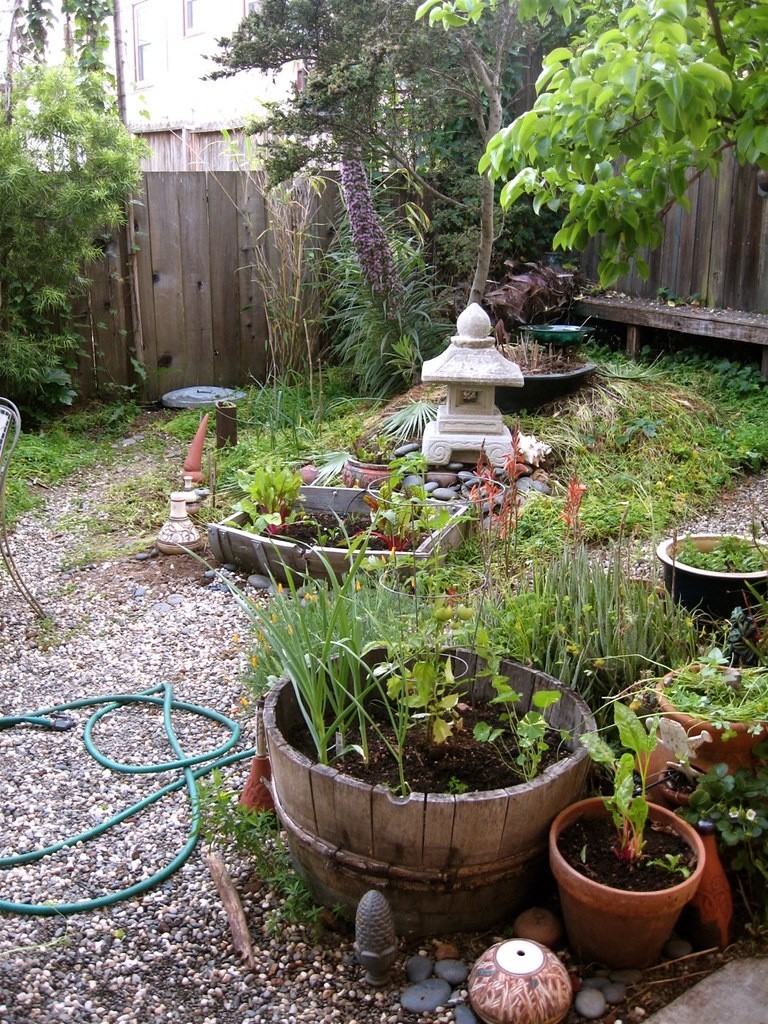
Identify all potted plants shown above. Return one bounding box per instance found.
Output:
[216,332,768,971]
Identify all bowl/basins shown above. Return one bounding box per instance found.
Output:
[518,324,595,346]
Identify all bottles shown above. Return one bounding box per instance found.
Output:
[156,491,201,554]
[182,476,200,514]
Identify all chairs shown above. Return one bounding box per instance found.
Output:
[1,395,46,620]
